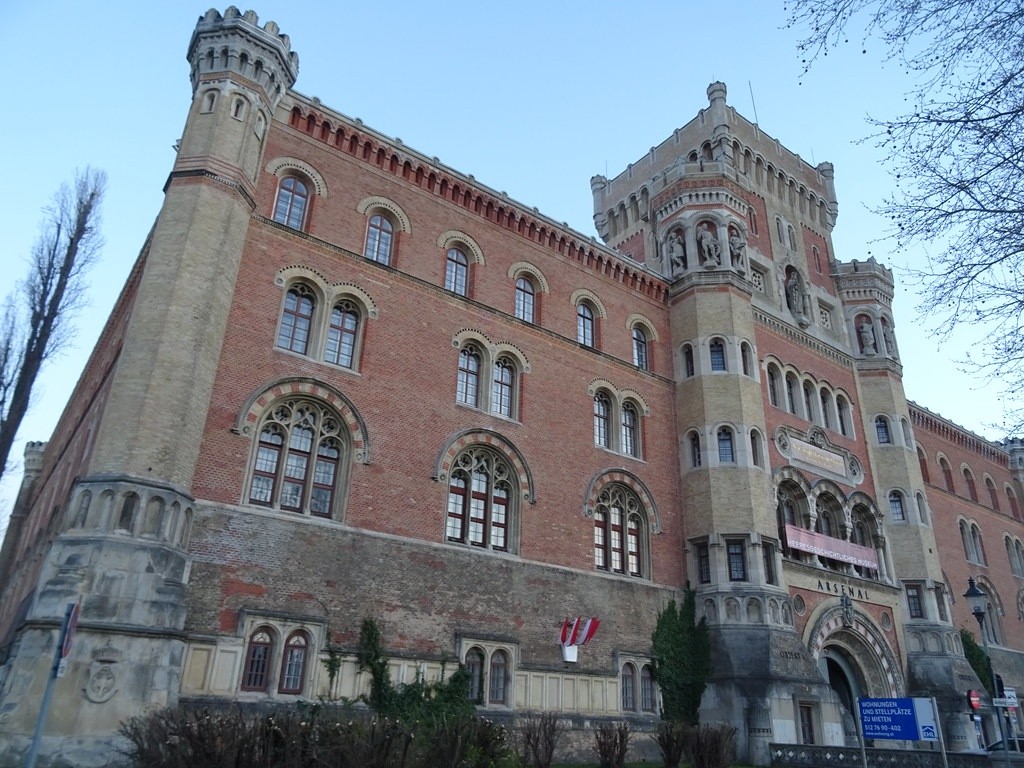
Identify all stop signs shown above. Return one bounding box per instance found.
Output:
[968,689,980,710]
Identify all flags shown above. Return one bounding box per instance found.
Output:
[557,615,601,647]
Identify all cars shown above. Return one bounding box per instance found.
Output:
[957,737,1024,754]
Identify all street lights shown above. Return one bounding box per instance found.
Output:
[961,574,990,656]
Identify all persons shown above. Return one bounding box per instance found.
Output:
[787,272,802,313]
[697,223,720,262]
[859,317,875,352]
[883,321,895,355]
[669,232,684,270]
[729,229,743,268]
